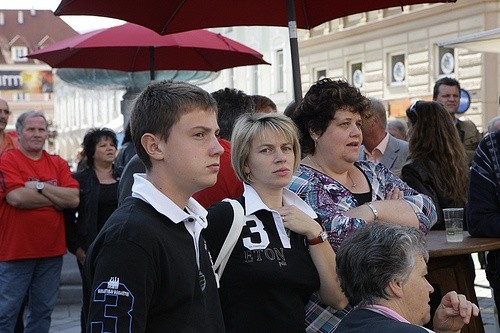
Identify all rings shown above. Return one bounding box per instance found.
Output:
[282,215,285,222]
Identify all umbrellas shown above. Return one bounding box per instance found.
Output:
[25,22,271,83]
[53,0,457,103]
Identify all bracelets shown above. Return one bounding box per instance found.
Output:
[365,202,378,220]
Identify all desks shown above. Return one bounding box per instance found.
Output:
[426,231,500,333]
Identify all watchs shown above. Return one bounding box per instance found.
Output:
[308,232,330,245]
[36,181,45,193]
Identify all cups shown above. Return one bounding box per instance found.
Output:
[443,208,464,242]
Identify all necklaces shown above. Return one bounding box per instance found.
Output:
[348,174,356,187]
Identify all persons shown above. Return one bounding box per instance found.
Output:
[65,126,121,277]
[0,110,80,333]
[80,80,224,333]
[113,120,148,206]
[0,99,20,156]
[193,76,500,333]
